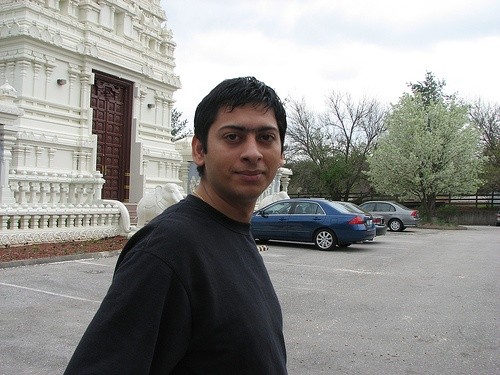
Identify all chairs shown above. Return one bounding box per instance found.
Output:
[295,206,315,214]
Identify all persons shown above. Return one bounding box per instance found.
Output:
[63,76,288,375]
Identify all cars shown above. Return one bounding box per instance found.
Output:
[247,197,377,251]
[357,201,425,233]
[333,201,387,237]
